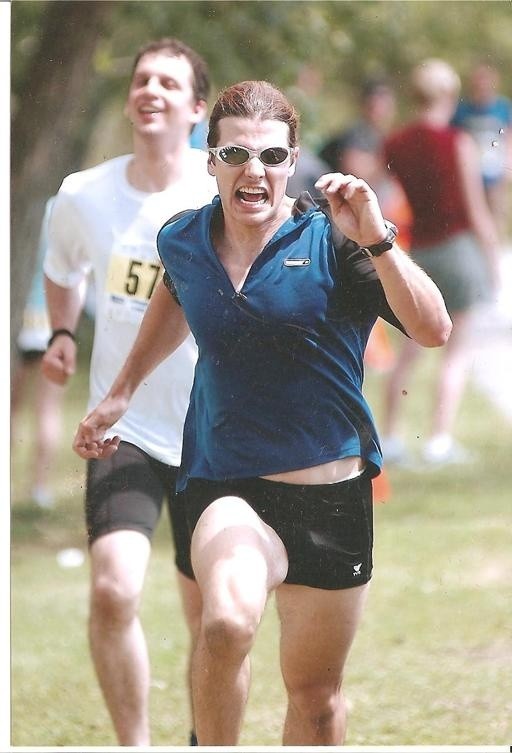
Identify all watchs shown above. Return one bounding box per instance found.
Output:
[358,215,399,259]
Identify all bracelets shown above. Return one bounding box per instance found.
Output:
[47,326,74,341]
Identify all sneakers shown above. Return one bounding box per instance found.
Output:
[385,433,489,476]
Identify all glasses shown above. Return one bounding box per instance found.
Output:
[207,144,299,167]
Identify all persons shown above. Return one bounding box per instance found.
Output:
[385,65,497,471]
[277,86,331,202]
[458,62,512,234]
[15,216,99,510]
[42,39,206,749]
[73,83,455,747]
[323,67,393,367]
